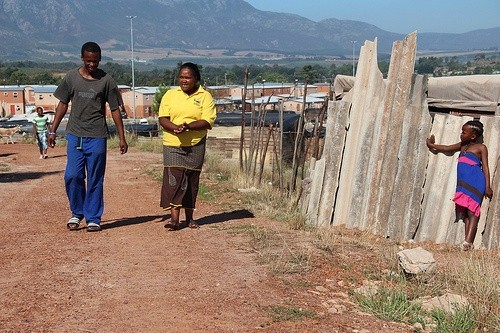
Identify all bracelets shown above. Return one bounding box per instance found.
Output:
[49,132,55,134]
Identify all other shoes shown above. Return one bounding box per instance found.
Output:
[456,240,474,251]
[44,155,48,159]
[40,155,44,159]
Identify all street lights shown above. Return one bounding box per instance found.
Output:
[126,15,138,120]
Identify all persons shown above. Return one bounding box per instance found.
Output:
[48,42,128,233]
[32,106,51,158]
[427,120,493,251]
[158,63,216,230]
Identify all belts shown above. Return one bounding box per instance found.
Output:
[77,137,82,150]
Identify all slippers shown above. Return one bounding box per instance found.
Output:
[87,223,101,231]
[67,218,81,230]
[187,221,199,229]
[164,219,180,229]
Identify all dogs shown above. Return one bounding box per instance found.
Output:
[0,125,22,144]
